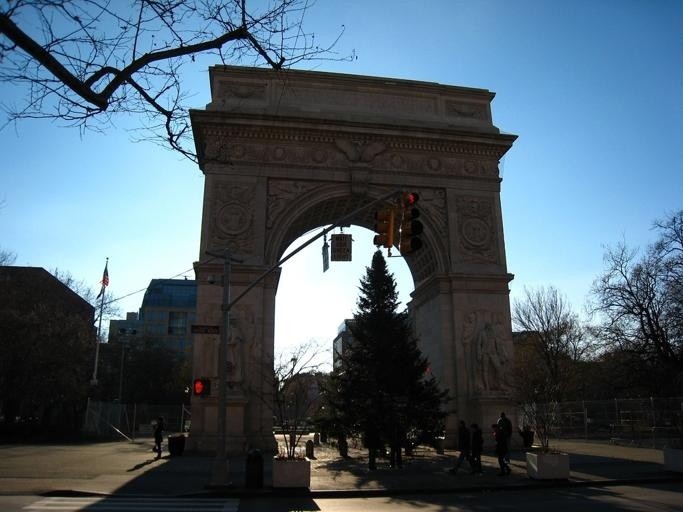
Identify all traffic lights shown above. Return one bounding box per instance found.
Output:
[373,193,422,256]
[194,377,211,396]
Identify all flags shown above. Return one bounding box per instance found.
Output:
[98,262,109,286]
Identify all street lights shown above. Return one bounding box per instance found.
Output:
[117,328,137,403]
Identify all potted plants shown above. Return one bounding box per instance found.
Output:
[492,298,592,481]
[235,354,330,489]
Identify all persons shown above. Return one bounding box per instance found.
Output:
[202,309,255,390]
[151,414,165,459]
[363,410,535,478]
[461,309,511,393]
